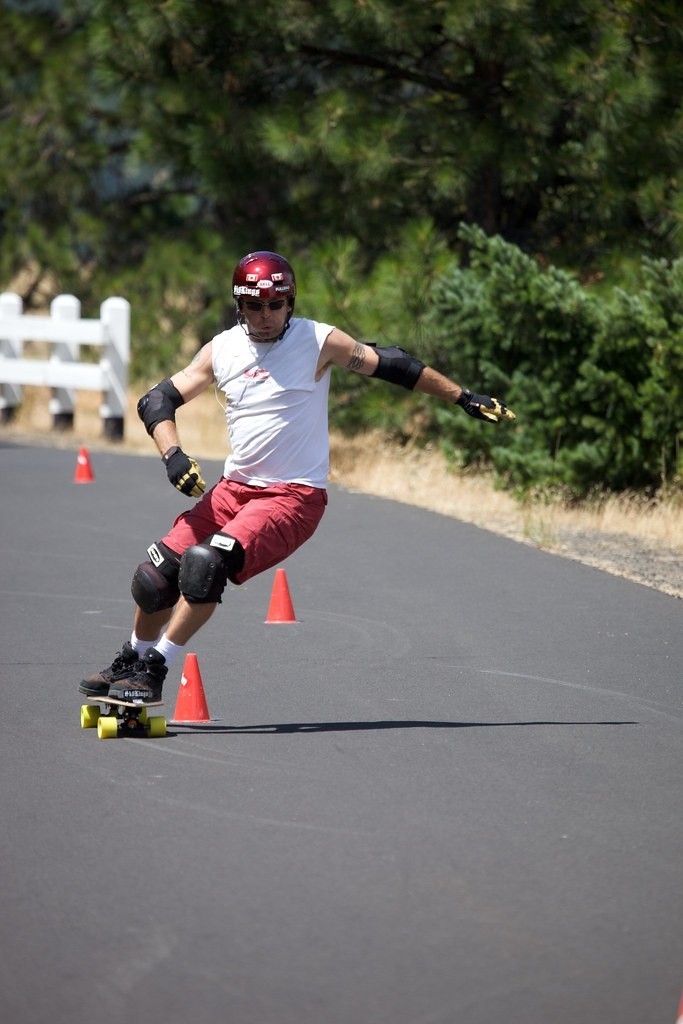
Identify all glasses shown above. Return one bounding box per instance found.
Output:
[240,299,286,311]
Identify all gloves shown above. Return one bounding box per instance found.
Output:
[161,446,206,498]
[453,387,517,424]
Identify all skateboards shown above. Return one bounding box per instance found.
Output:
[79,694,168,740]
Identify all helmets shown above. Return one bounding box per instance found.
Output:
[232,251,296,300]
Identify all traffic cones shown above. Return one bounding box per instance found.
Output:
[169,651,222,724]
[72,445,97,486]
[262,568,302,624]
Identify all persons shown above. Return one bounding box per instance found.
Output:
[78,251,517,703]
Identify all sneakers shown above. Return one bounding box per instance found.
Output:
[108,647,168,701]
[78,642,140,697]
